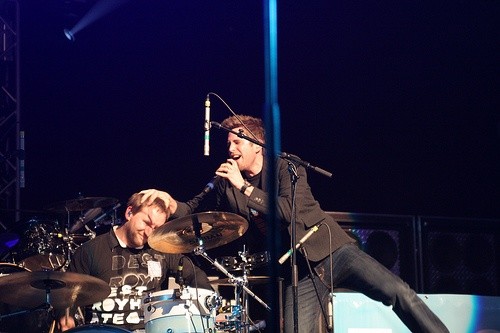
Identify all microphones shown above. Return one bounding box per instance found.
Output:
[175,257,184,286]
[79,217,96,240]
[204,100,211,155]
[278,225,319,264]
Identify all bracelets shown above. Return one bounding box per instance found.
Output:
[240,180,252,193]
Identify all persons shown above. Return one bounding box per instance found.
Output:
[137,115,448,333]
[52,192,238,333]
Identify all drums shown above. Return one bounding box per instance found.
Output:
[5,218,69,271]
[141,285,221,333]
[57,323,135,333]
[213,250,272,271]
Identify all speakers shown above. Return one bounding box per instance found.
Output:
[322,212,418,294]
[418,215,500,297]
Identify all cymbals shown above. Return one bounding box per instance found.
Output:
[0,269,111,311]
[147,211,249,254]
[44,197,119,212]
[209,274,285,285]
[65,231,90,242]
